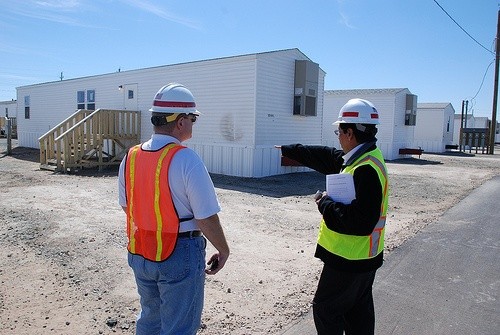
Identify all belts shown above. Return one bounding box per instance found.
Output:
[178,231,205,238]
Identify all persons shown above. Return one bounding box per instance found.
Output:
[274,97,390,335]
[118,83,230,335]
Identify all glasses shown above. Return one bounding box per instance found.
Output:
[184,116,197,122]
[334,129,348,135]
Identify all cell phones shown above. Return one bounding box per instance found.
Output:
[313,189,323,200]
[211,257,218,271]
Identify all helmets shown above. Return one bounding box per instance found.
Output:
[332,98,380,125]
[148,82,201,114]
